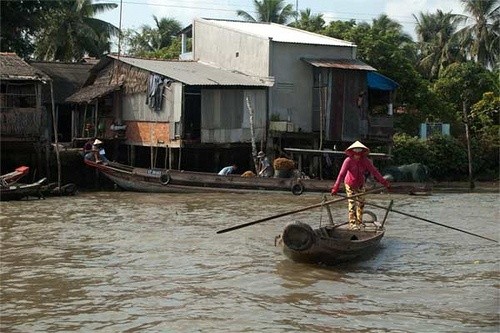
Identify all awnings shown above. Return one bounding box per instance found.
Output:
[367,70,400,90]
[302,58,378,71]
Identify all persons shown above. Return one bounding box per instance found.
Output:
[332,141,390,230]
[256,150,272,177]
[84,139,107,165]
[218,164,238,176]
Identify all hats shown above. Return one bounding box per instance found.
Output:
[257,151,265,158]
[345,141,370,156]
[94,139,103,145]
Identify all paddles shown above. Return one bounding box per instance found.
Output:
[215,188,393,235]
[332,193,500,244]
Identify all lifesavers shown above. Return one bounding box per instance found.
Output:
[158,173,171,185]
[282,220,315,252]
[361,209,378,223]
[290,183,304,195]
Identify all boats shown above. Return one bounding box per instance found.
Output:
[0,166,47,201]
[274,210,386,264]
[84,152,435,197]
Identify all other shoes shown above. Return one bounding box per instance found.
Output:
[96,160,103,164]
[352,225,359,230]
[359,223,366,228]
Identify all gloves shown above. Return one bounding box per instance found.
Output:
[331,188,337,196]
[385,182,392,191]
